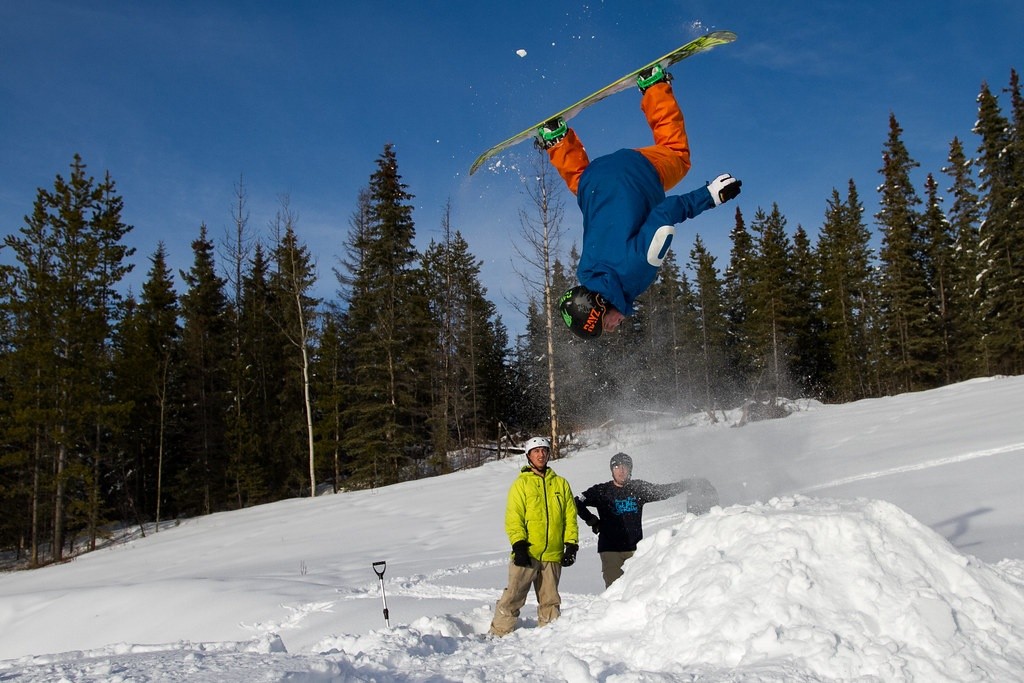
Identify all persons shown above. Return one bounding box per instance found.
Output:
[534,65,743,339]
[574,452,695,589]
[487,437,579,638]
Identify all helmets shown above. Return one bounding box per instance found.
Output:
[610,453,633,470]
[524,436,551,454]
[559,285,620,341]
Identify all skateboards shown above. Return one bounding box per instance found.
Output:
[468,19,740,178]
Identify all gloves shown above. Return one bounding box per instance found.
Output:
[585,514,601,535]
[560,542,579,567]
[512,540,533,568]
[707,174,742,208]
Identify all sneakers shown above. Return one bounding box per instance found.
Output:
[533,116,569,150]
[635,64,672,96]
